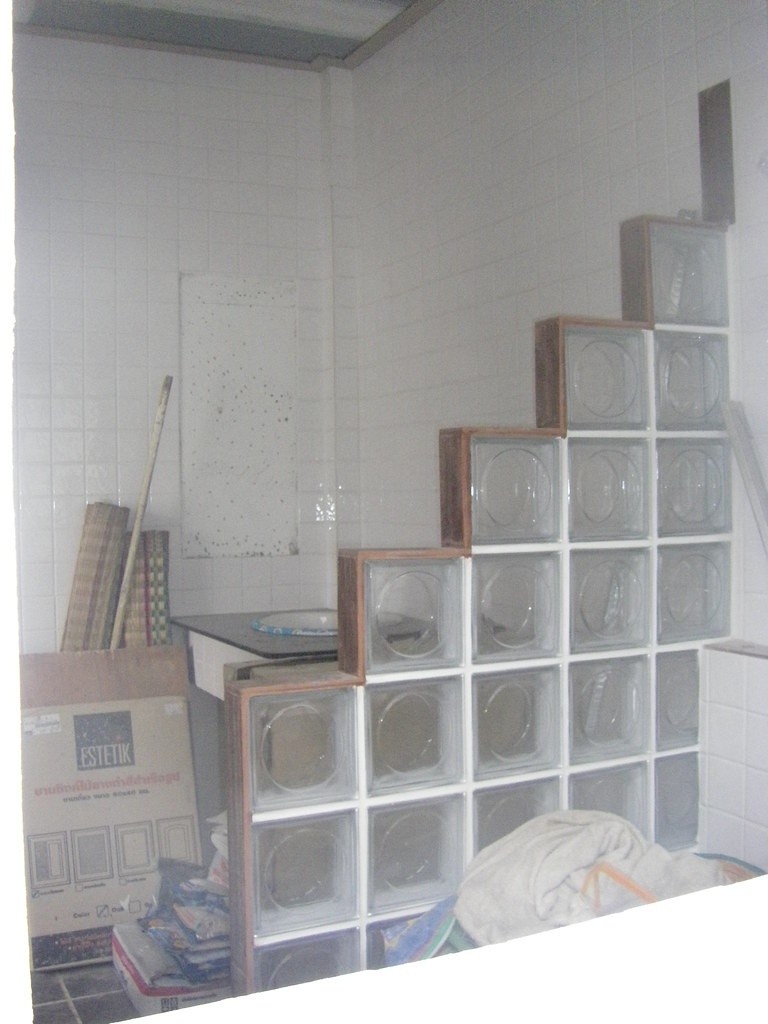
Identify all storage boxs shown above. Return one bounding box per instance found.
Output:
[111,924,234,1016]
